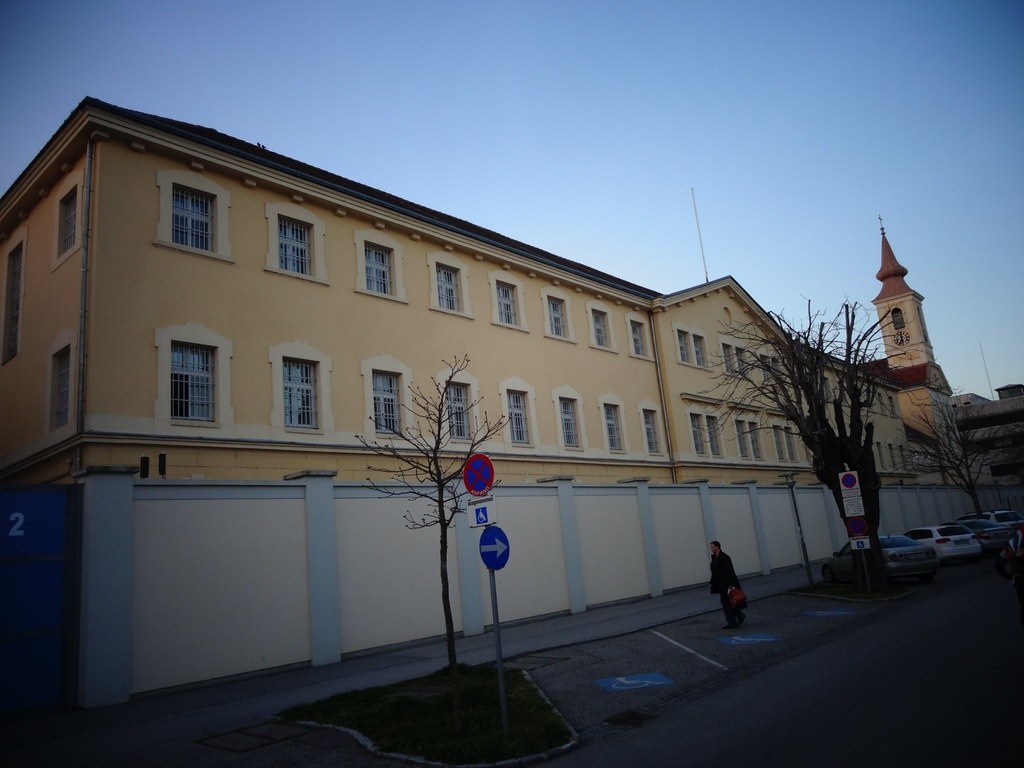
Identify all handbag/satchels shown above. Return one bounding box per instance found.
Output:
[728,586,747,609]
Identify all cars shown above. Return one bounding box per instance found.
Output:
[955,509,1024,541]
[895,525,982,565]
[948,518,1009,550]
[822,535,937,585]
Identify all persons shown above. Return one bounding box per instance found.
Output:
[995,523,1024,630]
[708,540,745,629]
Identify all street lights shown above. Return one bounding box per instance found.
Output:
[777,472,815,587]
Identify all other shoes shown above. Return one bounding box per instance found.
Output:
[722,623,740,629]
[738,614,745,626]
